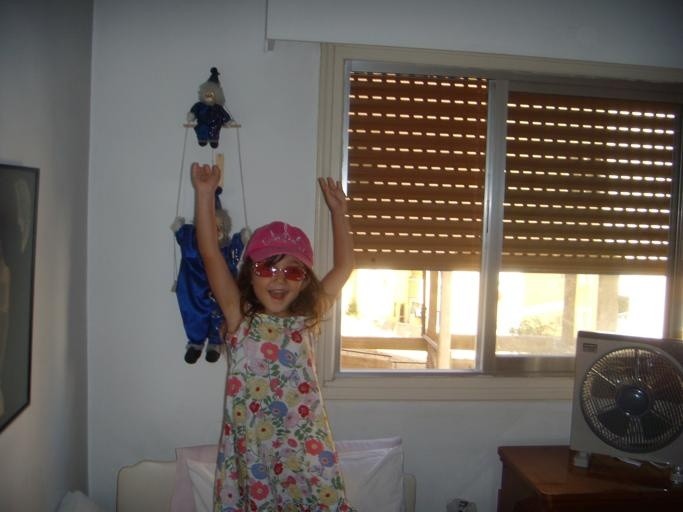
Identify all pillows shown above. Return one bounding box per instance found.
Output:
[168,438,409,511]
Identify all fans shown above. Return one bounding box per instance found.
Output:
[567,330,682,483]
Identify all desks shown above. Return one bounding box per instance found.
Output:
[497,443,682,511]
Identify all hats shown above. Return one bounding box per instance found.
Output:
[245,221,313,270]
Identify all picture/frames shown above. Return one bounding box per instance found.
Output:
[0,164,40,432]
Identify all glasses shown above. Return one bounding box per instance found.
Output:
[253,262,305,281]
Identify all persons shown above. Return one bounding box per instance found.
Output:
[190,160,355,512]
